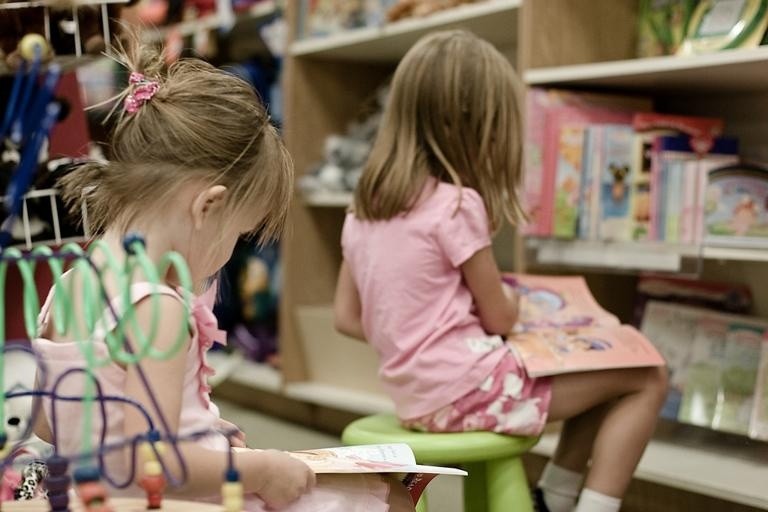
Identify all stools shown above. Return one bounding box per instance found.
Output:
[341,412,543,510]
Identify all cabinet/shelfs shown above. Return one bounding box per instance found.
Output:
[146,3,279,422]
[520,2,768,511]
[281,2,522,451]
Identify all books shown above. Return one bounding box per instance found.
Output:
[243,441,468,477]
[498,84,768,442]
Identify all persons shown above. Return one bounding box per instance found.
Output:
[31,42,412,512]
[334,29,666,512]
